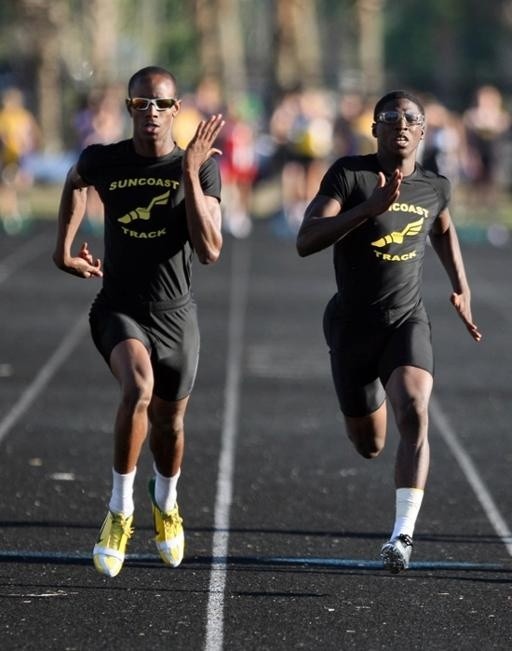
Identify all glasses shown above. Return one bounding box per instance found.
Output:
[374,110,424,125]
[126,96,177,112]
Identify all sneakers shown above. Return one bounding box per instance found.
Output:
[145,477,186,568]
[378,532,415,576]
[92,502,136,580]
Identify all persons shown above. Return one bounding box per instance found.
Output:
[0,73,256,251]
[238,78,511,250]
[295,88,482,576]
[53,65,227,579]
